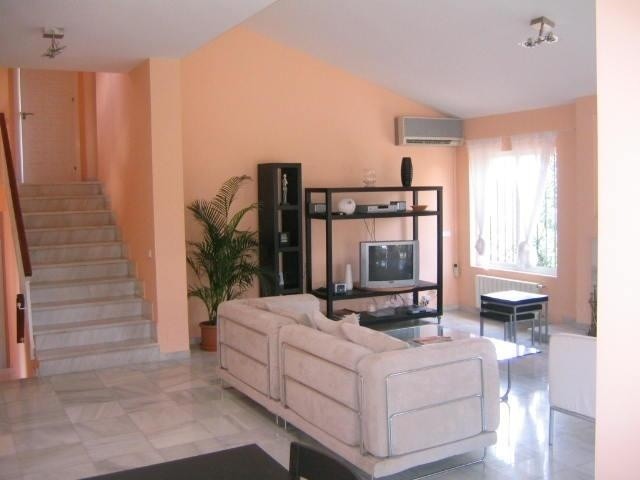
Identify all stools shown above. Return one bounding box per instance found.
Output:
[481,312,535,345]
[482,304,544,345]
[481,290,549,355]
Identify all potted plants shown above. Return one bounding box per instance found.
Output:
[187,174,258,351]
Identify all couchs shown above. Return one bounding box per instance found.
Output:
[216,294,500,480]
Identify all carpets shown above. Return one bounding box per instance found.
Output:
[79,442,290,480]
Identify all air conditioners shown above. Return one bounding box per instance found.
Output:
[398,116,464,145]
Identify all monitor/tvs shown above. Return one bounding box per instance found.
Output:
[359,240,419,288]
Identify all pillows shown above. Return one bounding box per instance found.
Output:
[266,301,316,329]
[312,310,358,339]
[341,322,409,354]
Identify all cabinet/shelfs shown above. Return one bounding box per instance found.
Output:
[257,163,303,297]
[305,186,443,324]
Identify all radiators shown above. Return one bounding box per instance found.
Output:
[474,275,543,321]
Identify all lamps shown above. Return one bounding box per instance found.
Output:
[39,26,66,59]
[523,17,558,48]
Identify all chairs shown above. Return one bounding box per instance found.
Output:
[290,440,360,480]
[548,332,597,446]
